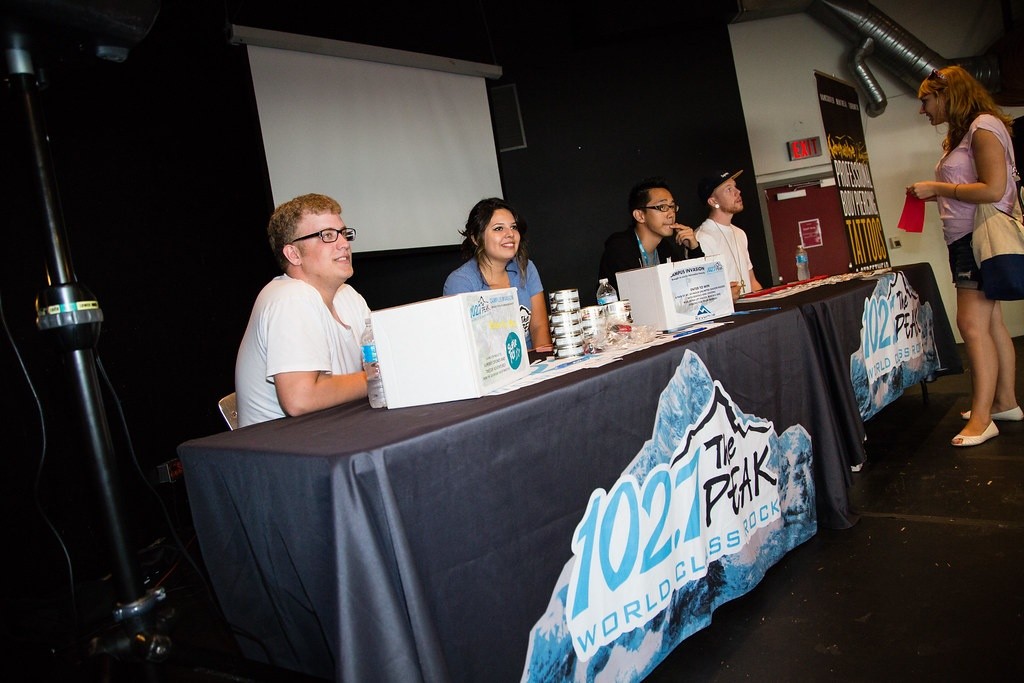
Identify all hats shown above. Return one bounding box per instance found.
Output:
[699,165,744,205]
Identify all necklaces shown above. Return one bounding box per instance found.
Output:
[708,216,748,296]
[634,233,658,270]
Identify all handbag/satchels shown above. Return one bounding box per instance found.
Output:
[969,198,1024,300]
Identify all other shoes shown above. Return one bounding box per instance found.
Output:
[950,421,999,446]
[961,406,1023,421]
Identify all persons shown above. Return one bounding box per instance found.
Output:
[595,180,705,304]
[442,197,551,352]
[233,193,373,428]
[682,169,763,297]
[906,65,1024,447]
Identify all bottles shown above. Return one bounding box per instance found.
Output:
[596,277,623,327]
[796,244,810,281]
[359,318,388,409]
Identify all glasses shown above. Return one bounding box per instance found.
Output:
[927,69,947,80]
[639,203,680,213]
[289,226,357,243]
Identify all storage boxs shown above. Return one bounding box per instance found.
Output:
[613,253,737,336]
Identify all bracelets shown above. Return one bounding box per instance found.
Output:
[954,183,961,202]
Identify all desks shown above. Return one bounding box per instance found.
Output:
[169,259,965,683]
